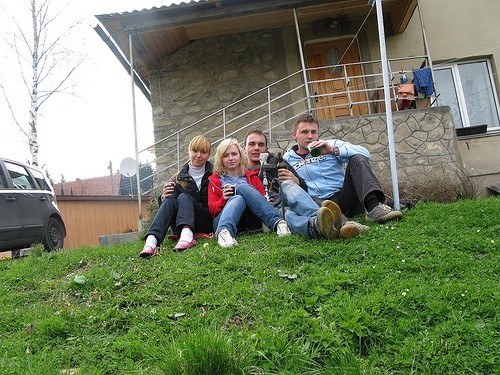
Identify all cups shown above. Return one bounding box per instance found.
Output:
[229,185,236,197]
[168,182,176,199]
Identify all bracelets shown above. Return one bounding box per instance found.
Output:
[331,146,338,155]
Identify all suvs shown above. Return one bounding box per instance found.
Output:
[0,157,67,253]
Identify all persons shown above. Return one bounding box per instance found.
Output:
[140,112,404,259]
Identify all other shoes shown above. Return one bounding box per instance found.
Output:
[174,238,196,250]
[140,246,160,257]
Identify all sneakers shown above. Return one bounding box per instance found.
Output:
[340,220,370,239]
[276,220,292,238]
[365,202,403,221]
[321,201,342,237]
[217,229,238,248]
[310,208,340,238]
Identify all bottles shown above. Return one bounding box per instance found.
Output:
[277,151,289,183]
[307,142,320,157]
